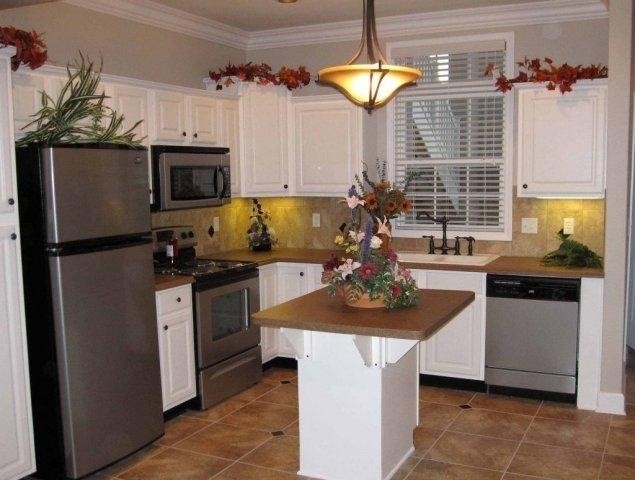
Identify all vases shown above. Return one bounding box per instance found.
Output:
[327,268,399,311]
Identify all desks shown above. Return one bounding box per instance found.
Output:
[246,280,477,480]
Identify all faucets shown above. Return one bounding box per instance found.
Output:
[416,211,450,255]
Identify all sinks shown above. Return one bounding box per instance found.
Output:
[399,256,489,263]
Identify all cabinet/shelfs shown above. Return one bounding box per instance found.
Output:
[305,262,336,295]
[398,266,489,394]
[6,54,389,206]
[0,52,24,220]
[505,77,612,200]
[256,258,280,374]
[0,222,41,480]
[154,276,202,418]
[273,259,305,370]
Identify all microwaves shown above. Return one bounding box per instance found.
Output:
[149,144,232,211]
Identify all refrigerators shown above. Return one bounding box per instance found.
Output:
[16,142,166,479]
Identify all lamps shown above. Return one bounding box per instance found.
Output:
[312,0,425,118]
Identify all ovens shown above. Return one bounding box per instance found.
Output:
[190,277,264,411]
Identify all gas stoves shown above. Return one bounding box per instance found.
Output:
[157,258,258,280]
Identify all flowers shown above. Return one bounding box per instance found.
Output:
[314,149,429,312]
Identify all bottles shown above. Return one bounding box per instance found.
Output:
[166,232,181,278]
